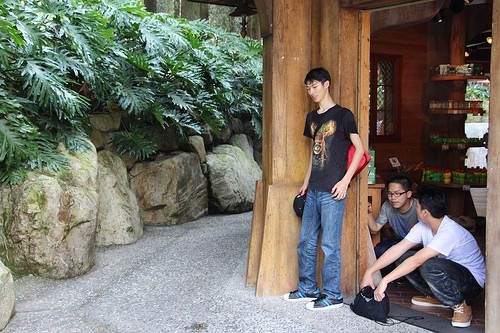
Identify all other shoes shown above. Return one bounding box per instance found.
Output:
[391,277,409,284]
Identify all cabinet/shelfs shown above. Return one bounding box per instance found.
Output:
[423,73,490,189]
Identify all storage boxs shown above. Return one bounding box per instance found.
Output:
[368,148,376,185]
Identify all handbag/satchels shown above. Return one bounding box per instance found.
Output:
[345,142,371,180]
[293,194,307,217]
[349,286,389,323]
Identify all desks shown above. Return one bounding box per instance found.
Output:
[367,183,385,248]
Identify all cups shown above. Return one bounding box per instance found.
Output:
[439,64,450,77]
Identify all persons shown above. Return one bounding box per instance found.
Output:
[282,67,365,312]
[360,189,486,328]
[368,175,420,284]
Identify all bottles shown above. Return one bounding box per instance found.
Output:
[430,130,441,143]
[429,99,483,113]
[415,166,487,186]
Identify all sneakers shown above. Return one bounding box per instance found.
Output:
[452,300,473,327]
[306,293,344,311]
[411,296,452,308]
[283,290,321,302]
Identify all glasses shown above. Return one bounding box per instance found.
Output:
[385,190,407,197]
